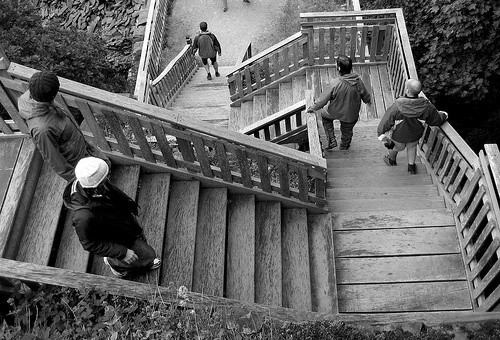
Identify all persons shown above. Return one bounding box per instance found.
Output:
[191,22,221,80]
[62,156,161,277]
[18,71,112,181]
[304,55,372,150]
[377,79,448,174]
[222,0,228,11]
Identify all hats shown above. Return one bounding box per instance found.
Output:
[75,157,109,188]
[28,71,60,103]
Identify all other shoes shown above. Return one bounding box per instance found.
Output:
[215,73,220,77]
[207,76,212,80]
[243,0,250,3]
[223,8,228,12]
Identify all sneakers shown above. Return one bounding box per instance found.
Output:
[339,144,350,150]
[408,163,417,174]
[325,139,338,149]
[151,258,161,269]
[104,257,127,277]
[383,154,397,166]
